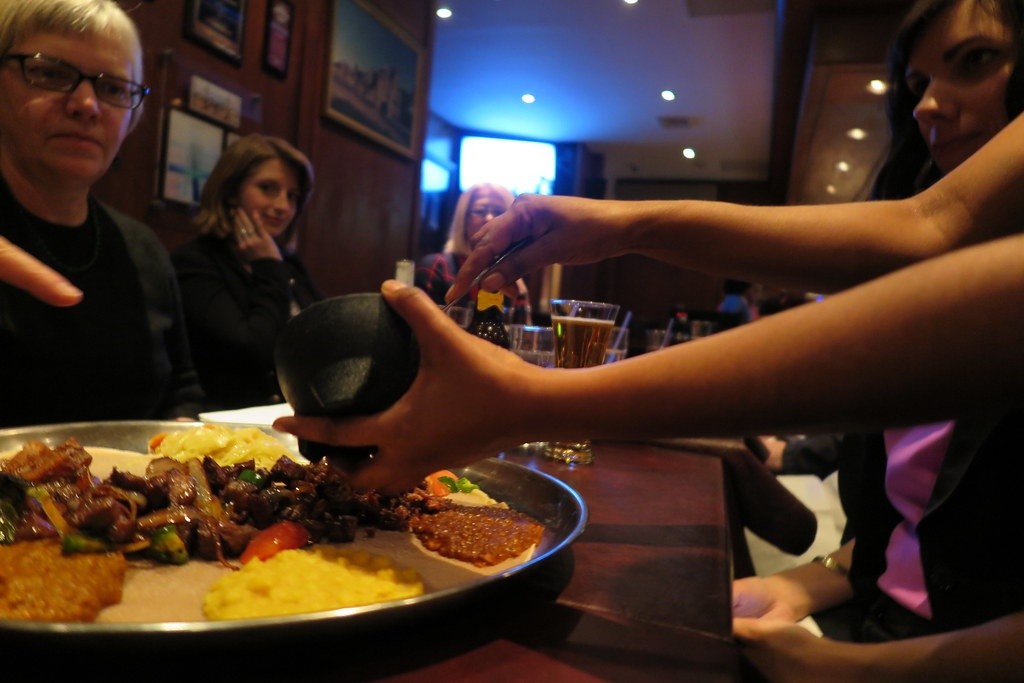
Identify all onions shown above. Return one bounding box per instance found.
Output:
[102,457,229,529]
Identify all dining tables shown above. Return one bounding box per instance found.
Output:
[379,437,734,683]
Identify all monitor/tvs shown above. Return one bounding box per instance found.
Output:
[460,135,558,198]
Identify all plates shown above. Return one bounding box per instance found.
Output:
[2,421,587,672]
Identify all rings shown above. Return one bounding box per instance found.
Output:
[240,228,247,237]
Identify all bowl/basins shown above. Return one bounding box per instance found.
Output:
[275,294,440,457]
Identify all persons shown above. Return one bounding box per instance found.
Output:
[414,183,534,351]
[0,0,337,426]
[272,0,1024,683]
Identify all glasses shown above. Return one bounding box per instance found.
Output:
[1,50,151,109]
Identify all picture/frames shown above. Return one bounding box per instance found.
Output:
[260,0,295,81]
[319,0,426,164]
[181,0,249,70]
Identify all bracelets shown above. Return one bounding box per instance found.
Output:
[812,555,849,578]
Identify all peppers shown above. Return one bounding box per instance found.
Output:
[0,468,483,564]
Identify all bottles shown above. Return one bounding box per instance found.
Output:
[465,284,509,354]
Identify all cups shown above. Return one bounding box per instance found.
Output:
[503,295,720,464]
